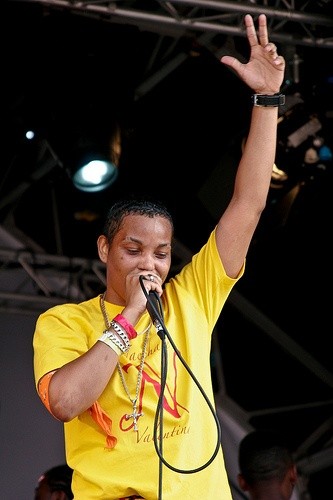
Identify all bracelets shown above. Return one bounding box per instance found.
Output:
[112,313,138,340]
[103,329,128,354]
[109,320,132,349]
[250,91,286,108]
[97,334,123,356]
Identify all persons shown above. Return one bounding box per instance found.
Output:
[238,428,297,499]
[33,464,74,500]
[31,13,285,499]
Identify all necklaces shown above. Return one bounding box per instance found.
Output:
[100,293,151,432]
[99,289,153,339]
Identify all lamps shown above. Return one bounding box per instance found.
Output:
[240,111,332,192]
[36,127,118,193]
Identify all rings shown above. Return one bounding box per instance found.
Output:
[269,45,277,54]
[148,274,154,282]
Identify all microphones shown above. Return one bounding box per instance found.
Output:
[145,291,164,340]
